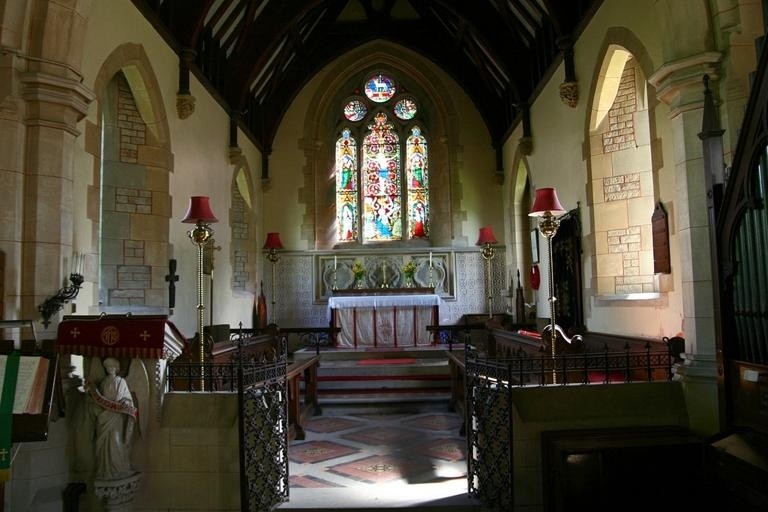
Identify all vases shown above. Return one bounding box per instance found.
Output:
[400,277,423,288]
[346,275,370,289]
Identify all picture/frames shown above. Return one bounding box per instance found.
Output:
[529,227,539,263]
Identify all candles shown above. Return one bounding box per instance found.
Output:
[334,255,337,270]
[75,250,86,274]
[382,260,386,281]
[429,252,433,267]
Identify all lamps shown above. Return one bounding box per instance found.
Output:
[180,195,220,391]
[262,233,285,326]
[474,226,499,320]
[528,187,569,384]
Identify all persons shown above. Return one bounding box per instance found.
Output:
[85,356,138,482]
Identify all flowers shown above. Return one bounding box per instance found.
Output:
[351,259,368,280]
[403,261,417,279]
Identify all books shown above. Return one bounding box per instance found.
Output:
[0,355,49,418]
